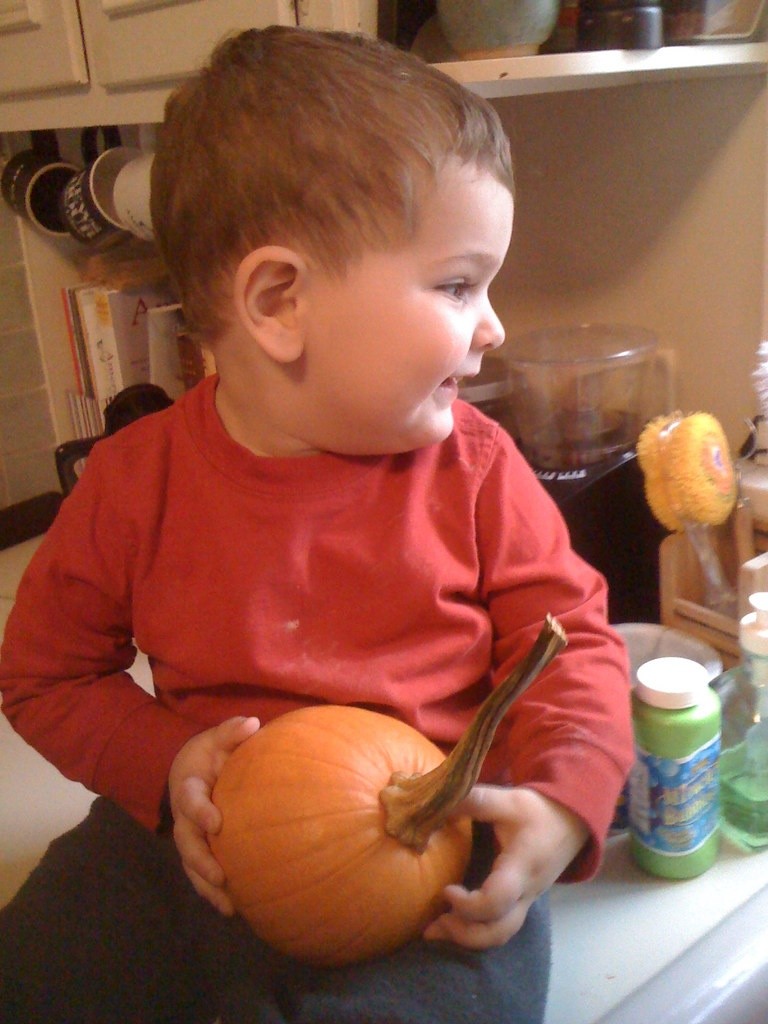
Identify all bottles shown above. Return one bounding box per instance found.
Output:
[556,1,663,53]
[627,657,722,882]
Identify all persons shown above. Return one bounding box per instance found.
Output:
[0,26,635,1024]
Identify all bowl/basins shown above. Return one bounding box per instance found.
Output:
[503,321,657,471]
[436,0,559,61]
[607,621,721,688]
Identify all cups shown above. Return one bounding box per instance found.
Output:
[0,125,157,244]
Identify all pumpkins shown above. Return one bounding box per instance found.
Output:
[204,705,474,968]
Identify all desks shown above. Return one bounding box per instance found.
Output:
[0,534,768,1024]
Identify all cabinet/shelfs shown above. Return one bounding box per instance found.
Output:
[0,0,768,136]
